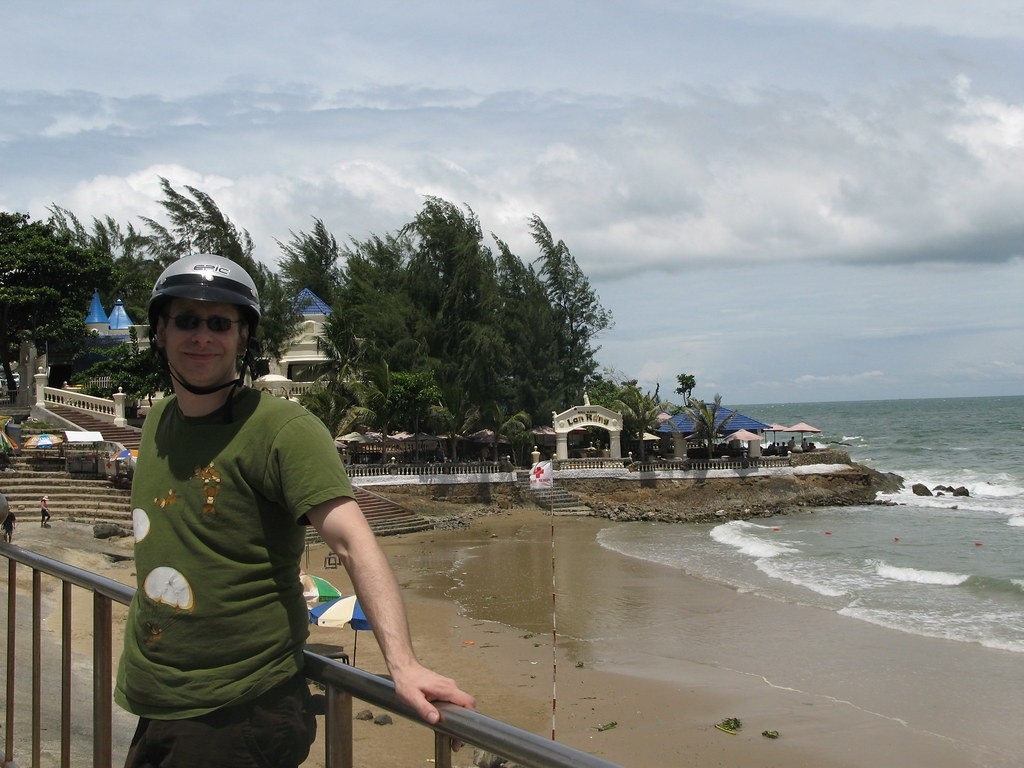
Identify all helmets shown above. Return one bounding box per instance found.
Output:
[148,253,261,337]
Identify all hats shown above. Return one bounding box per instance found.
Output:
[43,496,50,501]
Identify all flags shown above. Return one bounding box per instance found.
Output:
[529,461,553,491]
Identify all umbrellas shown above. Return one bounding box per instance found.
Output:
[783,422,822,445]
[528,424,556,445]
[436,430,511,462]
[724,429,763,448]
[401,431,435,455]
[25,431,62,458]
[653,410,672,423]
[763,423,788,445]
[387,429,415,451]
[630,431,661,447]
[111,447,139,475]
[0,427,21,472]
[299,571,375,670]
[761,422,770,443]
[335,428,396,455]
[684,431,723,447]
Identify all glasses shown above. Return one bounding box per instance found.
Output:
[168,312,240,332]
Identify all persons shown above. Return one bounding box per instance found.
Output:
[113,253,477,768]
[780,442,788,455]
[40,495,51,528]
[788,436,798,451]
[774,442,783,455]
[1,507,16,544]
[801,438,816,451]
